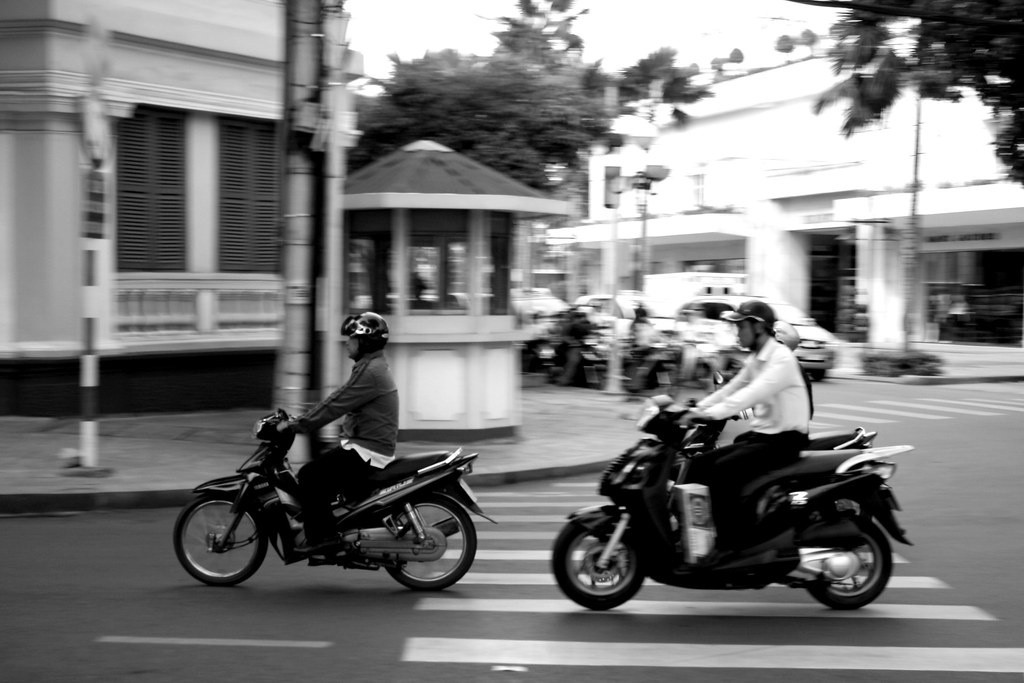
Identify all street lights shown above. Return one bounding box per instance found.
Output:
[600,132,670,293]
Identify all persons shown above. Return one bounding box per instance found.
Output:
[680,300,811,564]
[277,311,399,563]
[547,302,719,380]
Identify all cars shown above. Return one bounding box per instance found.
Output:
[674,293,840,383]
[358,286,623,341]
[921,281,988,325]
[946,293,1024,347]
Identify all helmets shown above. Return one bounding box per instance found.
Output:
[341,311,389,353]
[773,321,799,351]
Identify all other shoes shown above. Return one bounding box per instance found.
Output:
[705,549,739,570]
[294,532,341,553]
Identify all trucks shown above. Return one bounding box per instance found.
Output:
[641,274,750,317]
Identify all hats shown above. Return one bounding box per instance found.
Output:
[721,300,775,330]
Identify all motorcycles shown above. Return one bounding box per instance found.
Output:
[521,323,613,386]
[552,389,915,617]
[171,405,499,591]
[617,329,682,394]
